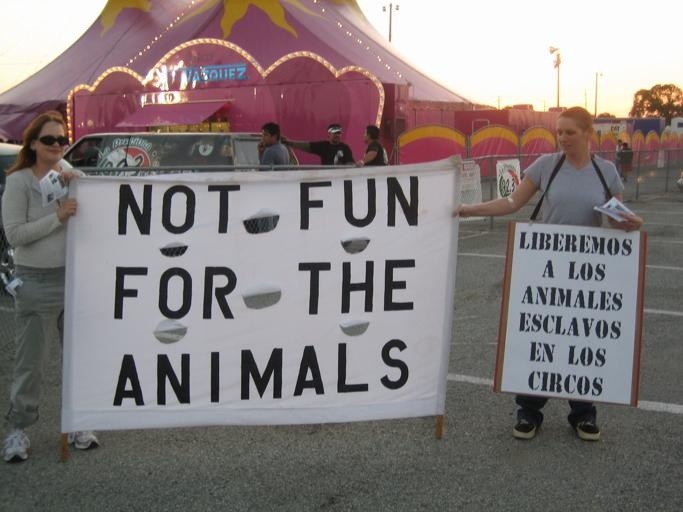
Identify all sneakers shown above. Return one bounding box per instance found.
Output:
[3,430,31,463]
[512,419,537,440]
[575,420,601,442]
[67,431,101,450]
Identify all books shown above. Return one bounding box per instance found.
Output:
[592,195,635,223]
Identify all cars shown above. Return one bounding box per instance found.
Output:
[63,132,301,256]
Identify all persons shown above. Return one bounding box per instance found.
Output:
[356,125,389,167]
[453,106,643,441]
[281,124,355,165]
[0,111,100,463]
[615,139,634,182]
[258,123,290,171]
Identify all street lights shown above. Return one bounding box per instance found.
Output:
[548,46,561,107]
[595,71,603,118]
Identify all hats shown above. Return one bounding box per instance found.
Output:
[328,127,345,135]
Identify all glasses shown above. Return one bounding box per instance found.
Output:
[34,135,69,147]
